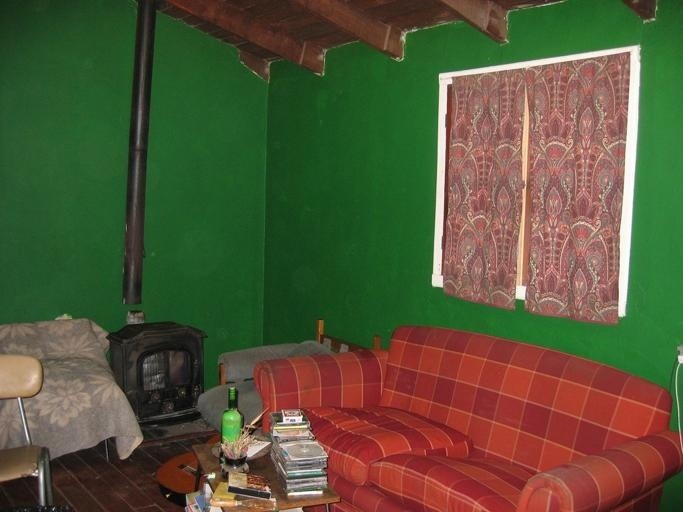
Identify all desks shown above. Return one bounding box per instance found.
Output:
[190,440,343,512]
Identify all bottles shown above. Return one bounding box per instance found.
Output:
[220,385,244,442]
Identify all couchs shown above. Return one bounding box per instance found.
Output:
[253,320,683,512]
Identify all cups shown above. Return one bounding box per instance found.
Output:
[219,451,246,480]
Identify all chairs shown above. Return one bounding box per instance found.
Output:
[2,353,59,512]
[0,316,140,473]
[193,321,382,438]
